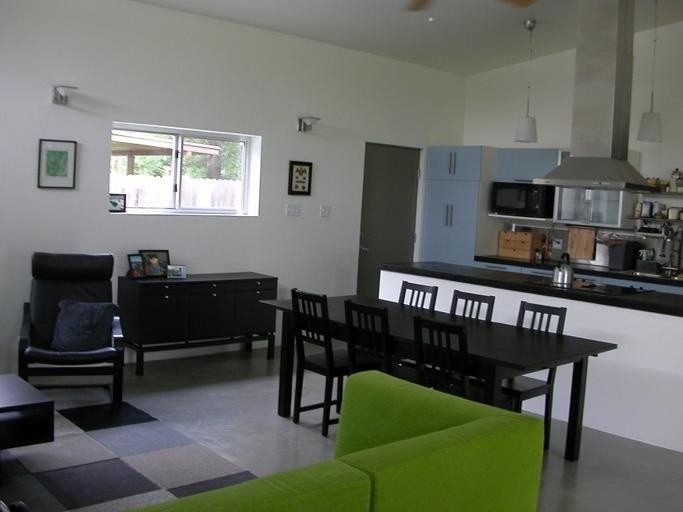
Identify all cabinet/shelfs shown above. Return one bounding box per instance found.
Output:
[495,146,564,186]
[633,200,667,219]
[645,169,683,194]
[626,188,683,224]
[534,248,540,262]
[416,143,502,266]
[114,270,280,378]
[581,189,592,224]
[679,207,683,220]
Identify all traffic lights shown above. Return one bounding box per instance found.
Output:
[45,296,116,352]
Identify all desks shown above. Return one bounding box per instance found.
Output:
[257,293,617,463]
[0,372,55,451]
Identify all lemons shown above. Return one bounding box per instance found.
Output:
[549,252,575,292]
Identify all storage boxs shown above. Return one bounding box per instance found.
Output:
[498,230,545,262]
[608,241,645,270]
[567,226,596,260]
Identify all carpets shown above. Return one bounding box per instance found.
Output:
[0,399,261,512]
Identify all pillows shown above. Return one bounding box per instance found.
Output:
[45,296,116,352]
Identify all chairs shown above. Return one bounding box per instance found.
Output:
[468,301,566,414]
[450,290,495,321]
[14,249,126,409]
[398,281,438,310]
[289,287,350,437]
[343,300,415,381]
[412,315,512,407]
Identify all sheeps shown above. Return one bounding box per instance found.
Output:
[658,225,683,277]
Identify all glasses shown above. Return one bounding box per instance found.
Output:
[49,82,80,106]
[634,1,665,143]
[298,112,322,133]
[512,20,542,144]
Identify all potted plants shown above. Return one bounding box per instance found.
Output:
[287,159,312,197]
[126,253,144,280]
[108,192,127,213]
[35,138,77,191]
[138,249,171,279]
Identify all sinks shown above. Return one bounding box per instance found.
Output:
[118,365,549,511]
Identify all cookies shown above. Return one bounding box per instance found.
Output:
[489,177,556,219]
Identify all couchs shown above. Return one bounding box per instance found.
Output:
[118,365,549,511]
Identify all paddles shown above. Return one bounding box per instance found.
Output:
[0,399,261,512]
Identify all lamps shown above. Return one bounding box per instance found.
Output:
[634,1,665,143]
[49,82,80,106]
[298,112,322,133]
[512,20,542,144]
[14,249,126,409]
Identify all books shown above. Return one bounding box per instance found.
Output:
[567,226,596,260]
[498,230,545,262]
[608,241,645,270]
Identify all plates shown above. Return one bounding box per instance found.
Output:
[416,143,502,266]
[495,146,564,186]
[114,270,280,378]
[626,188,683,224]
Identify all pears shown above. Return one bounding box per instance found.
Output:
[639,248,656,261]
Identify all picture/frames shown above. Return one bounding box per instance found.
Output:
[126,253,144,280]
[287,159,312,197]
[138,249,171,279]
[0,372,55,451]
[108,192,127,213]
[35,138,77,191]
